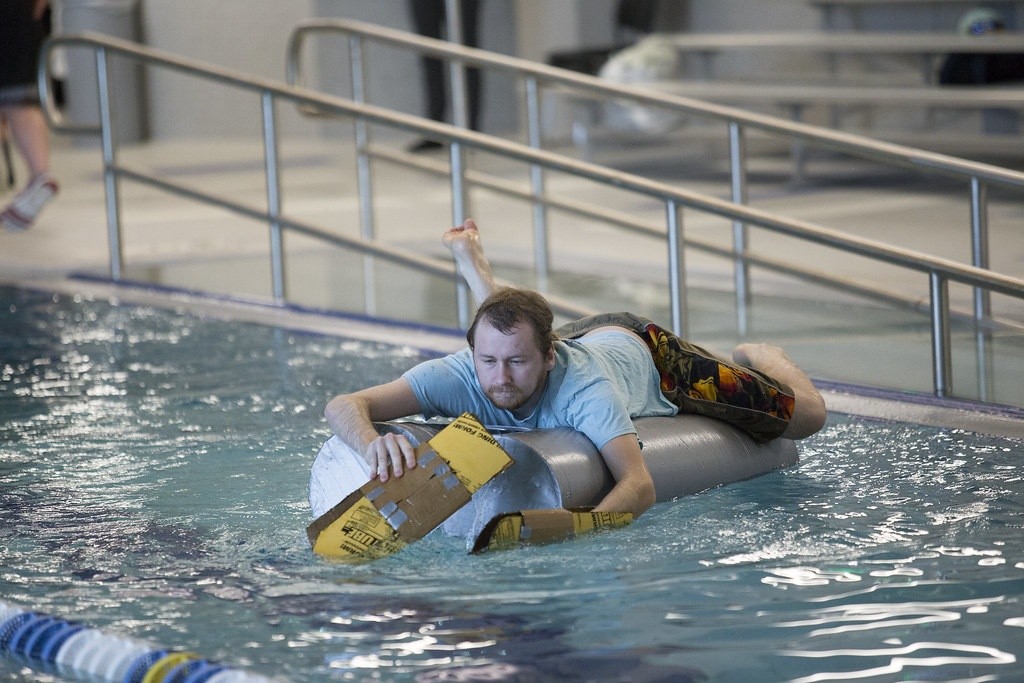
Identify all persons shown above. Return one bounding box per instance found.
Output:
[0,0,83,217]
[927,6,1024,127]
[321,213,828,540]
